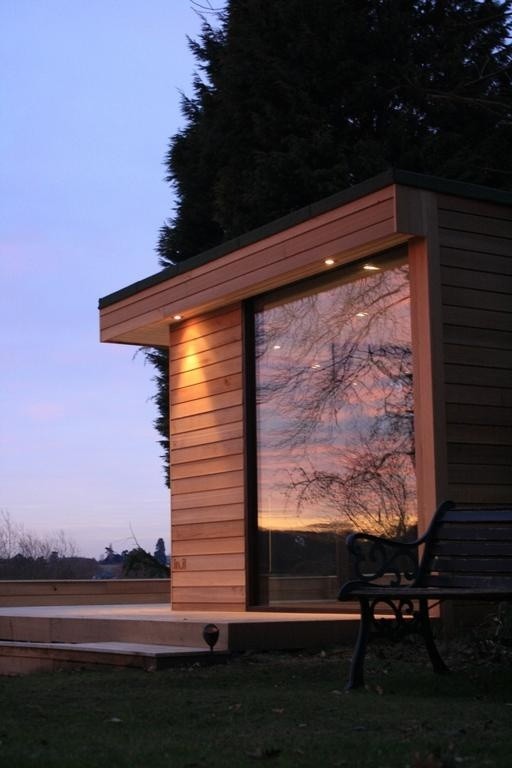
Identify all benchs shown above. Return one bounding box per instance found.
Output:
[338,499,511,687]
